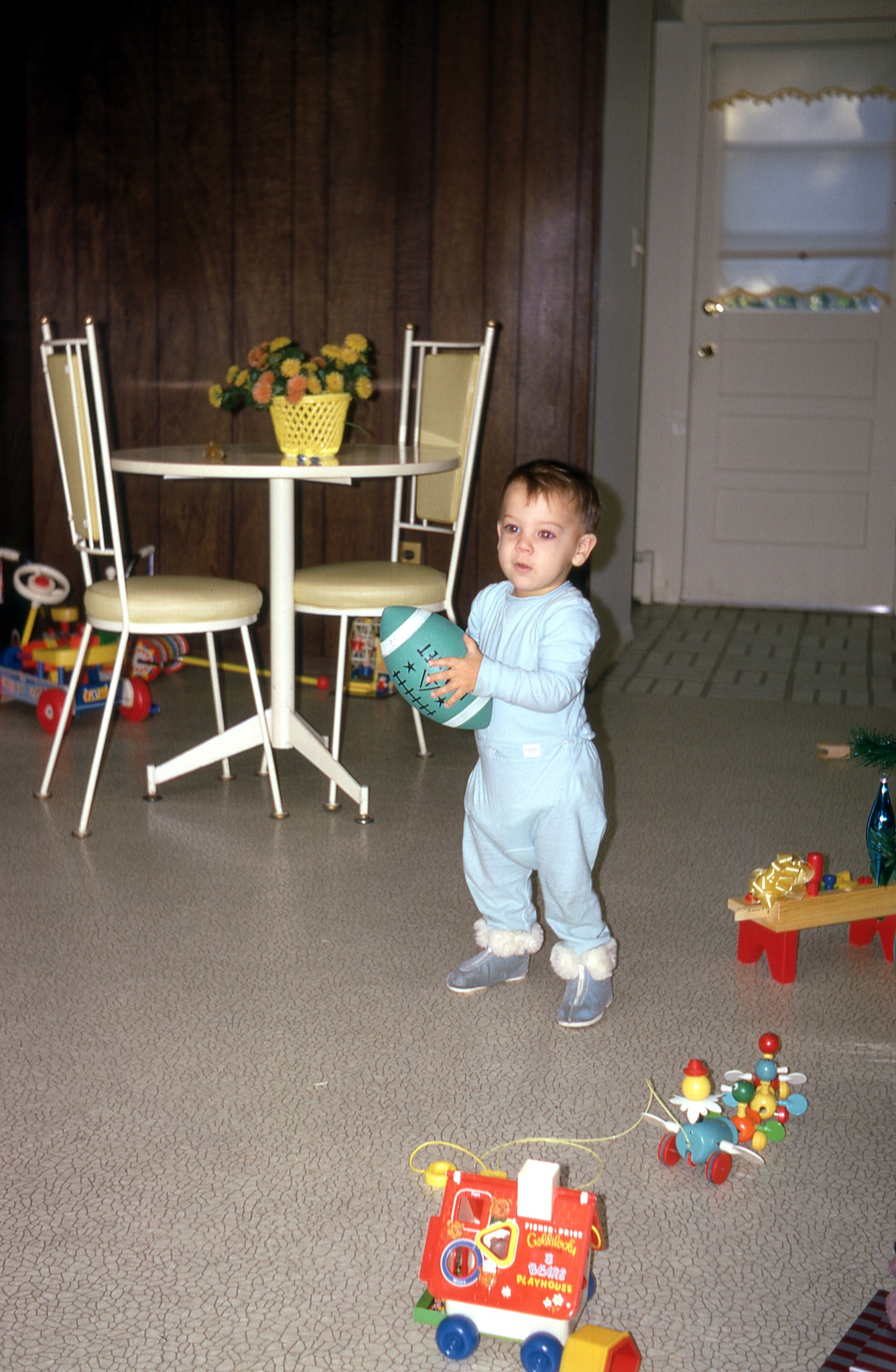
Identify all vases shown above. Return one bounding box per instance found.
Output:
[269,392,353,459]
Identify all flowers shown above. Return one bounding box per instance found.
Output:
[207,332,375,417]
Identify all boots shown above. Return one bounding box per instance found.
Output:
[549,936,619,1028]
[446,917,545,994]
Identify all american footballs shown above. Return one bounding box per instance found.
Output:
[381,603,494,732]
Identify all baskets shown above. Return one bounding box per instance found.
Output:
[268,391,353,458]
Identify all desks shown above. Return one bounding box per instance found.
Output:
[108,442,463,825]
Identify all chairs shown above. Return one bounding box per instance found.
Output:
[253,318,496,814]
[30,315,288,841]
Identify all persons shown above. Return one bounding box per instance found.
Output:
[427,459,616,1026]
